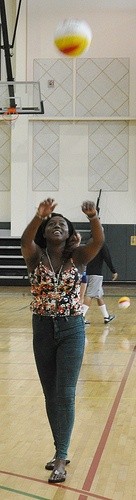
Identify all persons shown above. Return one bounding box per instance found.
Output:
[20,197,106,482]
[72,226,118,324]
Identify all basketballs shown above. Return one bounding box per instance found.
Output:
[53,18,93,58]
[117,296,131,310]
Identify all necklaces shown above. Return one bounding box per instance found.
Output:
[46,247,64,293]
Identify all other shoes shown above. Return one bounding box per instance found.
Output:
[83,317,90,324]
[104,315,115,324]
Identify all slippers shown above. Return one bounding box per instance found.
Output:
[45,453,70,470]
[47,466,67,482]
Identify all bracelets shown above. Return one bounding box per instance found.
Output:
[88,211,97,220]
[37,211,51,220]
